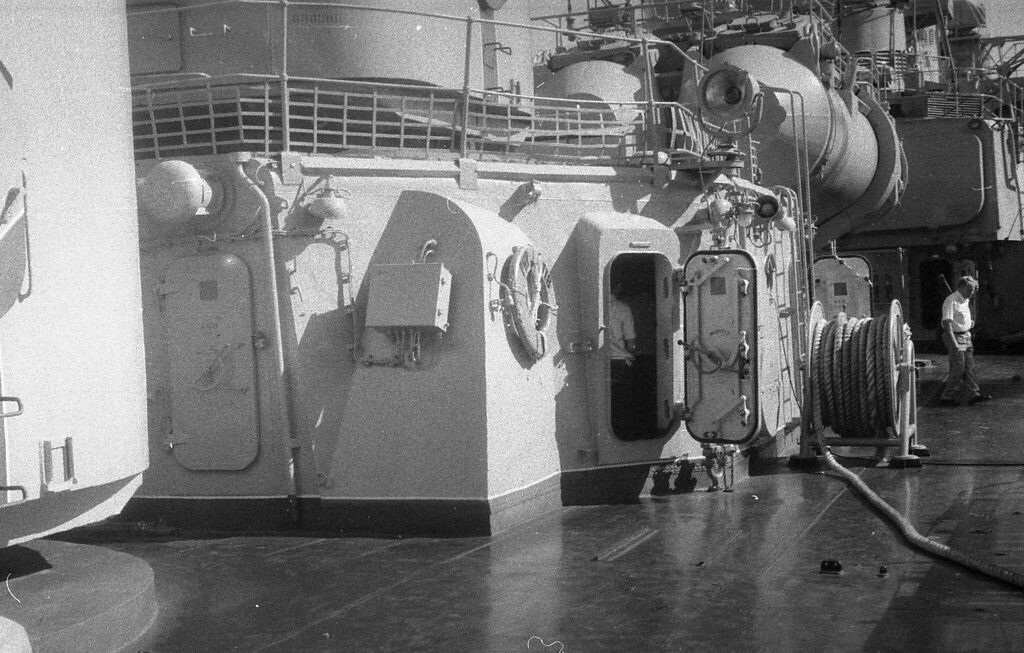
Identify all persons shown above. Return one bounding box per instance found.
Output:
[610,271,638,437]
[939,275,991,407]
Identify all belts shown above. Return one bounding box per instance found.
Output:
[953,328,972,335]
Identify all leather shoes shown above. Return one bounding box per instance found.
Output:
[941,394,963,406]
[968,393,993,406]
